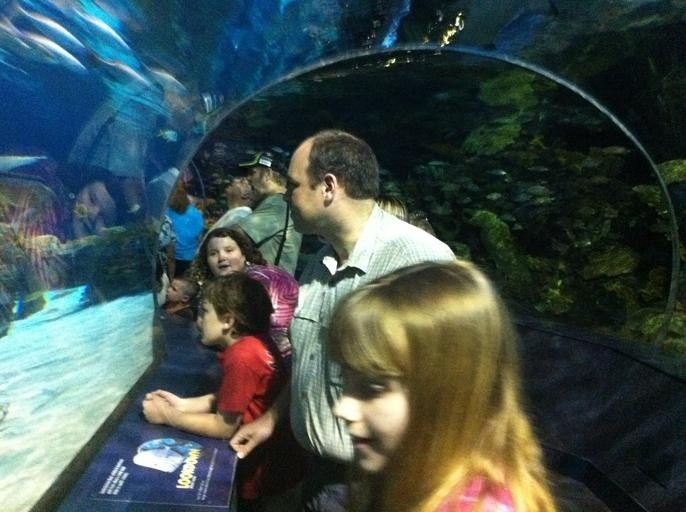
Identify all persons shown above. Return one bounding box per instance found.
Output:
[374,193,435,236]
[9,161,127,290]
[330,263,554,512]
[231,131,456,511]
[144,272,296,512]
[159,147,316,343]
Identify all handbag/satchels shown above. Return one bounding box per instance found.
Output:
[246,265,299,328]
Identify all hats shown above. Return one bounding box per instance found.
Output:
[239,146,287,173]
